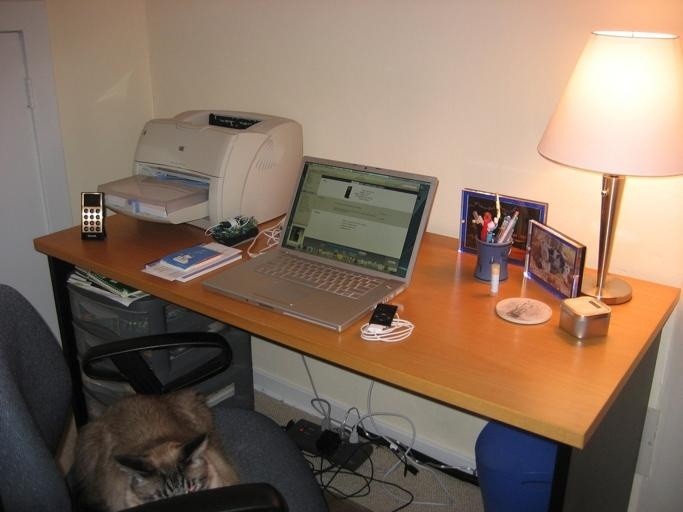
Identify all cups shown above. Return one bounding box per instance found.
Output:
[470,234,514,284]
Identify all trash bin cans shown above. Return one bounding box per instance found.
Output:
[475,421,556,511]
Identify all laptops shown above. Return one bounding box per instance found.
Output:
[201,155,439,334]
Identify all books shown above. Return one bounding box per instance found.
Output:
[67,242,243,308]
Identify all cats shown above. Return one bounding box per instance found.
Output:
[63,383,239,512]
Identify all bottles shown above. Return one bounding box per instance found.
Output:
[488,264,500,296]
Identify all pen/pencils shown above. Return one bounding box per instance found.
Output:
[473,193,520,243]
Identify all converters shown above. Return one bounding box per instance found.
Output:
[316,428,342,456]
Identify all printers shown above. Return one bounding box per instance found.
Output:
[97,108,303,231]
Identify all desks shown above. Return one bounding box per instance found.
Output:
[30,215,680,511]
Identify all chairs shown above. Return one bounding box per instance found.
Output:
[0,282,333,511]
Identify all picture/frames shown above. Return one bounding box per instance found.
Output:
[521,217,586,301]
[458,187,549,266]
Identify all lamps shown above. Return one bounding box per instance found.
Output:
[535,27,680,306]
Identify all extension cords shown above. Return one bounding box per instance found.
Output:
[283,419,374,472]
